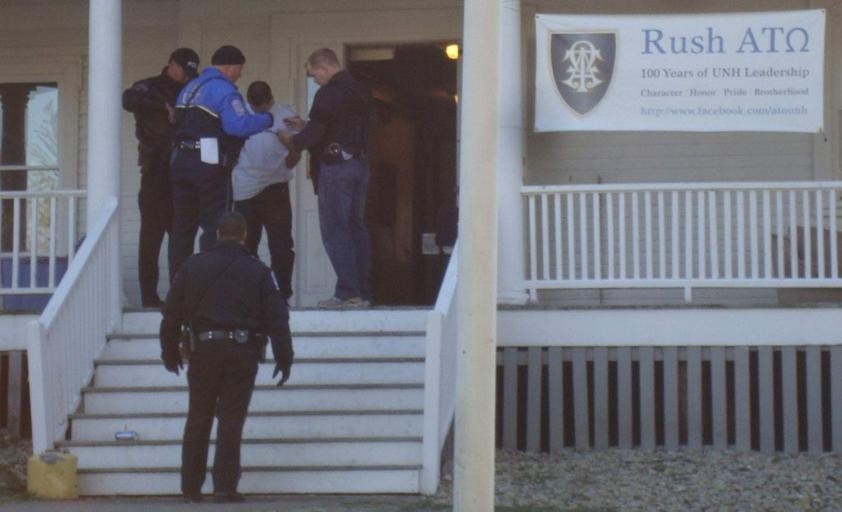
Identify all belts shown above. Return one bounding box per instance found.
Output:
[196,329,262,343]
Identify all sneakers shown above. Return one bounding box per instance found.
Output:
[213,487,247,503]
[316,296,375,311]
[141,294,166,308]
[183,489,205,503]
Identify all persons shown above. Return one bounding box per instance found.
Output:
[166,43,274,288]
[155,211,294,505]
[229,78,300,310]
[282,45,379,309]
[121,47,201,307]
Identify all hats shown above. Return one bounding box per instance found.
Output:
[168,47,200,79]
[211,44,247,66]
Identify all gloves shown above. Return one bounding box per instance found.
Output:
[270,360,293,387]
[160,353,185,376]
